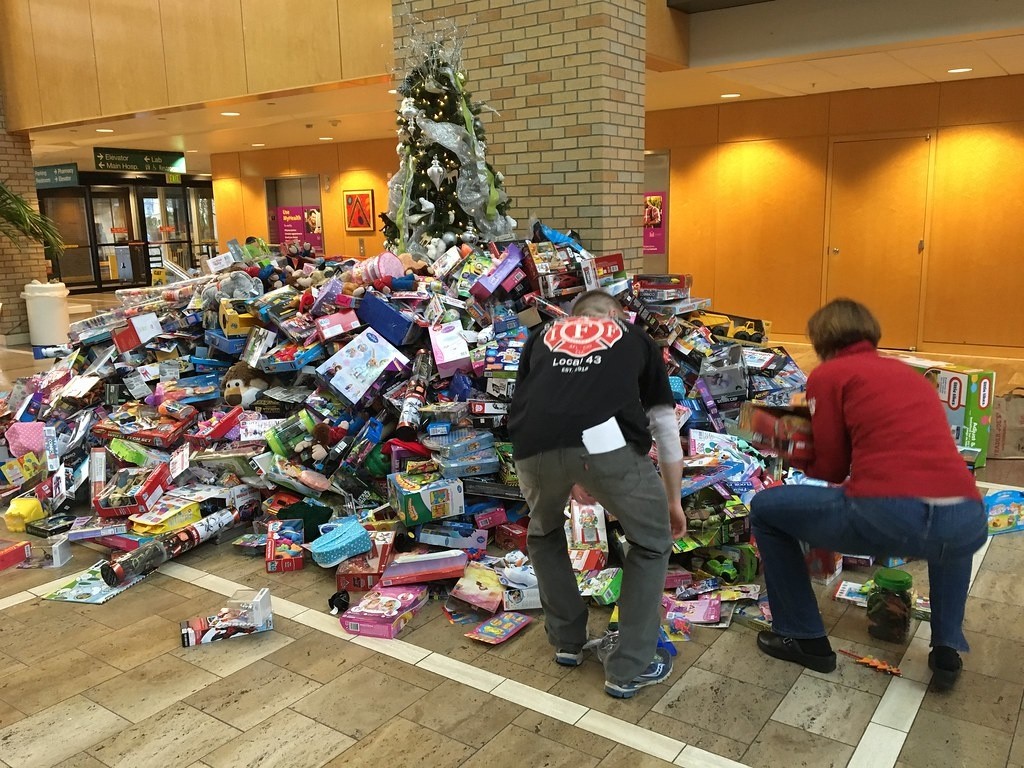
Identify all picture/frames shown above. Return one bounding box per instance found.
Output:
[343,189,376,232]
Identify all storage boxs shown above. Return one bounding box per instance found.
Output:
[0,239,1024,649]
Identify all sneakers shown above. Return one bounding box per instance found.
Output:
[604,645,674,699]
[555,625,590,668]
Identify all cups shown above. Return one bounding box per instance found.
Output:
[161,290,183,301]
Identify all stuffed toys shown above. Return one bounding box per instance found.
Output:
[221,360,282,410]
[201,244,434,310]
[295,406,371,460]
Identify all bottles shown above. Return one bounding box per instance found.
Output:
[865,567,917,643]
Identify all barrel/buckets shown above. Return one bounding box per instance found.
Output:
[352,251,405,284]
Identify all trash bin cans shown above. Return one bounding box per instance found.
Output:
[24,282,70,347]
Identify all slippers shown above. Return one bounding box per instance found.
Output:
[928,649,963,691]
[755,630,838,673]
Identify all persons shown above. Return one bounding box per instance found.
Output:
[747,297,988,689]
[507,291,686,698]
[305,210,318,233]
